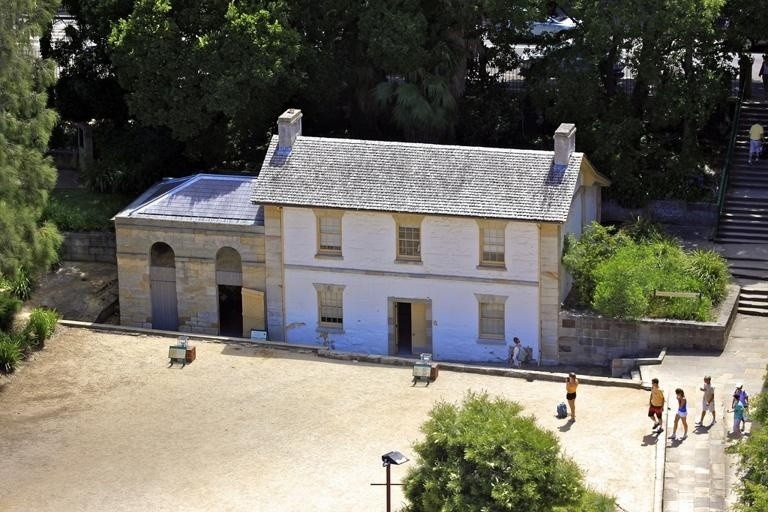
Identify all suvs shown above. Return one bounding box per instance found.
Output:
[725,1,768,50]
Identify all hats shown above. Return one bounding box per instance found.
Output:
[735,382,743,388]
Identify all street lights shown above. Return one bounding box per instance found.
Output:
[370,448,412,511]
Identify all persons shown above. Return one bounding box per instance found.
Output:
[668,389,688,440]
[565,373,579,422]
[746,117,765,165]
[647,378,665,434]
[694,376,717,425]
[727,394,745,441]
[732,384,748,431]
[758,54,767,92]
[512,336,523,367]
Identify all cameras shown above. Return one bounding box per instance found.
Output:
[567,377,570,382]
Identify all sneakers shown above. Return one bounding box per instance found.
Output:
[711,419,717,425]
[680,434,688,440]
[657,428,664,433]
[668,434,677,439]
[728,428,745,438]
[568,416,575,422]
[695,420,703,427]
[652,422,659,429]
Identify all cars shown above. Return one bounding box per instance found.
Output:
[518,38,585,72]
[488,15,584,47]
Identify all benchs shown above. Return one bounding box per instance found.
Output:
[508,346,532,372]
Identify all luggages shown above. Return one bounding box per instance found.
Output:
[557,401,567,418]
